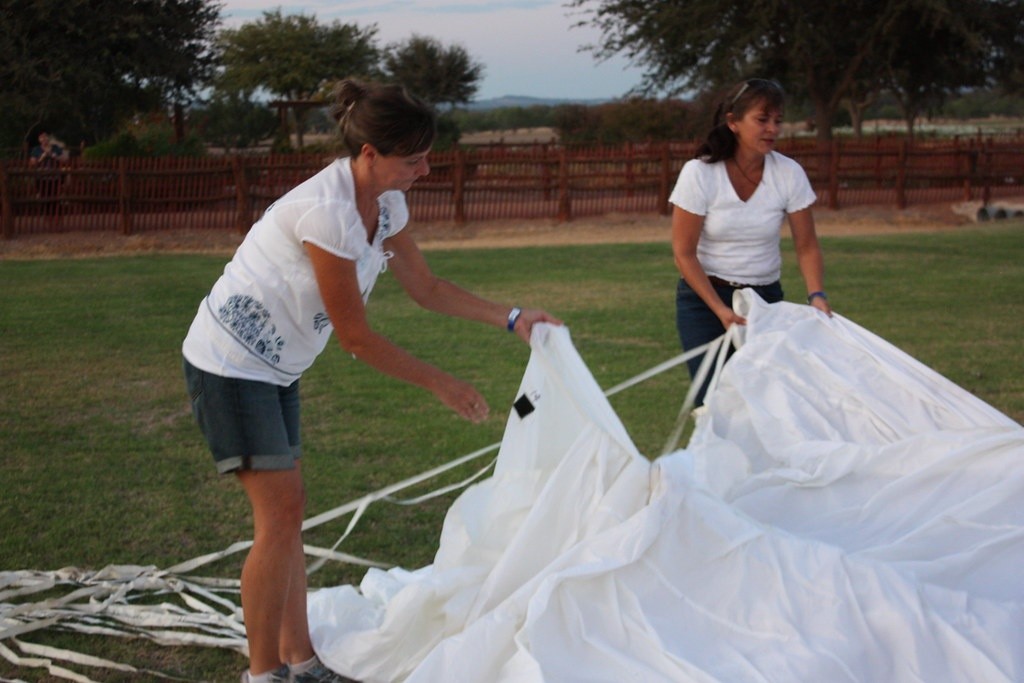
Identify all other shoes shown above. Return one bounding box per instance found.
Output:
[241,663,300,683]
[294,661,364,683]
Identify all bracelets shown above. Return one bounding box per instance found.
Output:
[506,306,520,332]
[806,291,826,305]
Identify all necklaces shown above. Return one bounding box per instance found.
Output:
[731,155,760,187]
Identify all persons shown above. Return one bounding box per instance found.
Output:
[666,72,833,409]
[29,131,67,217]
[78,140,91,156]
[181,78,563,683]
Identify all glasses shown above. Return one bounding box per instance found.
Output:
[726,79,782,111]
